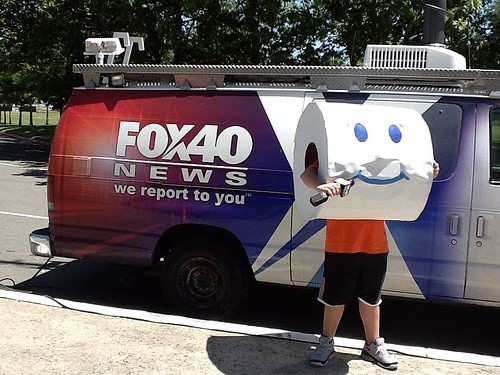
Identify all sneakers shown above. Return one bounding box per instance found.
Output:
[308,335,336,367]
[360,337,399,369]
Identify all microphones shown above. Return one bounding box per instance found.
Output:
[309,178,354,205]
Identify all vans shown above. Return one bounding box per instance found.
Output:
[29,32,500,310]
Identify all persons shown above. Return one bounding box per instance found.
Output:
[299,157,440,372]
